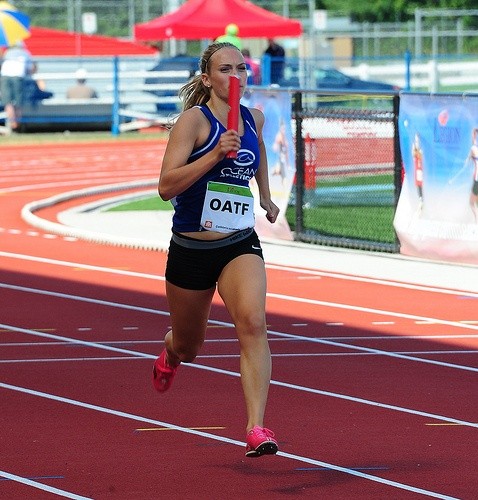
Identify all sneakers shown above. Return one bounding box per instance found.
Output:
[152,326,180,393]
[245,425,279,458]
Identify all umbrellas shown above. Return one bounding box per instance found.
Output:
[0,0,34,48]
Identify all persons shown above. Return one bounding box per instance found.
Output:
[465,129,478,220]
[7,61,98,133]
[240,39,286,84]
[269,122,292,188]
[412,135,426,209]
[153,42,280,457]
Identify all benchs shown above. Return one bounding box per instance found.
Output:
[15,100,115,133]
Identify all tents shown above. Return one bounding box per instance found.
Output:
[0,27,163,57]
[134,0,302,42]
